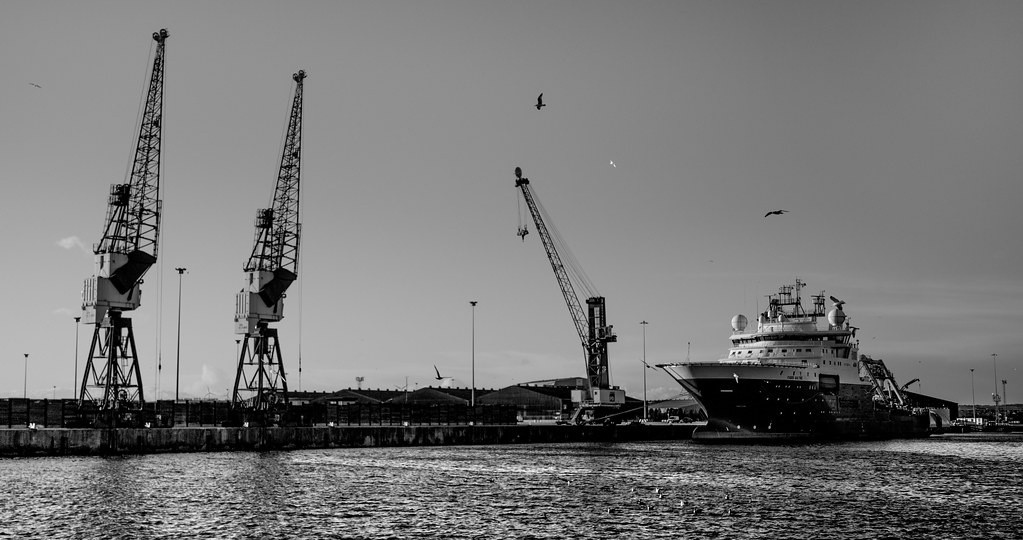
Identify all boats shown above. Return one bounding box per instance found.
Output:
[653,276,932,444]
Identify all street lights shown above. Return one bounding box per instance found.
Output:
[23,352,29,398]
[1001,379,1008,421]
[969,368,976,423]
[73,316,82,399]
[175,266,186,402]
[468,301,478,423]
[991,352,999,428]
[640,320,649,419]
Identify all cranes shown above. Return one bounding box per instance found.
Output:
[510,165,627,423]
[73,26,170,429]
[226,65,309,428]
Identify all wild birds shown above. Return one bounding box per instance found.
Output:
[609,160,616,168]
[764,210,791,217]
[565,478,796,522]
[534,92,547,111]
